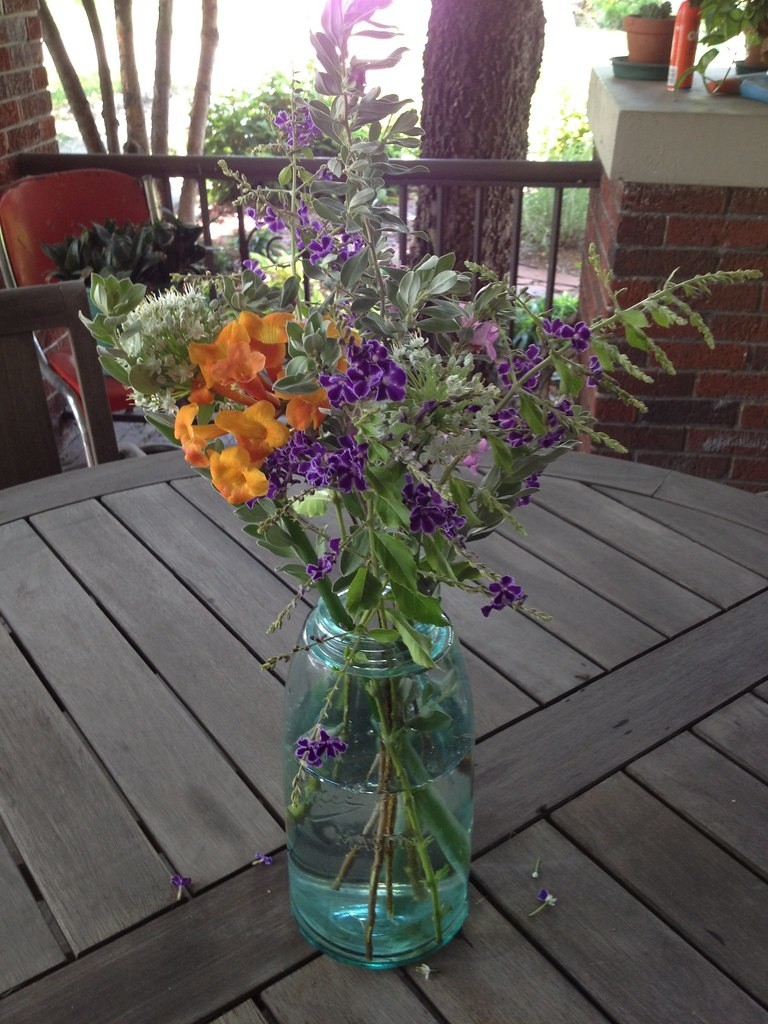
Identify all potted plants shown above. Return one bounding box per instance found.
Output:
[684,1,768,72]
[623,1,678,63]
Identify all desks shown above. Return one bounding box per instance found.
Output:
[2,439,768,1022]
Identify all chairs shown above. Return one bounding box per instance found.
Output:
[1,280,119,489]
[1,168,228,468]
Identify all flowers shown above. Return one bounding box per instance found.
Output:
[78,0,768,778]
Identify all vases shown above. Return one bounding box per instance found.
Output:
[284,588,476,970]
